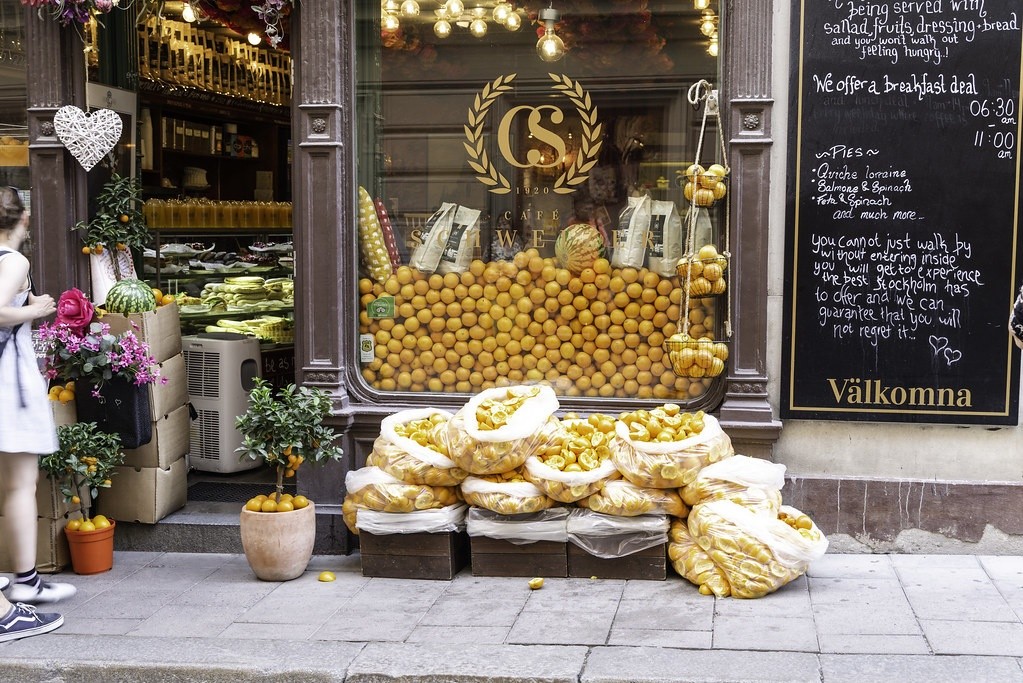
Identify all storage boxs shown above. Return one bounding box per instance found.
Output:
[111,403,190,470]
[147,349,190,421]
[97,453,188,524]
[96,299,183,365]
[0,400,92,572]
[161,116,259,158]
[358,525,469,581]
[255,170,273,189]
[254,189,274,201]
[567,527,669,581]
[469,526,569,578]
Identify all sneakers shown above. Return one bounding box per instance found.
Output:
[0,603,64,642]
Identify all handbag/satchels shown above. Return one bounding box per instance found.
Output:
[75,375,151,449]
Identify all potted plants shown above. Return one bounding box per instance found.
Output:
[38,420,126,576]
[232,376,344,582]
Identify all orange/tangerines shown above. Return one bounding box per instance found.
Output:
[83,215,129,254]
[283,438,320,478]
[66,514,111,531]
[358,244,727,399]
[245,492,308,512]
[72,455,111,504]
[47,381,75,404]
[97,288,175,318]
[684,163,727,205]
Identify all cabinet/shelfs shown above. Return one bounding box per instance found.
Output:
[142,95,276,201]
[138,227,295,410]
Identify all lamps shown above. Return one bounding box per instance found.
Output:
[536,0,565,63]
[381,0,521,38]
[693,0,718,57]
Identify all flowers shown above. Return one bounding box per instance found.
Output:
[20,0,120,28]
[38,288,170,402]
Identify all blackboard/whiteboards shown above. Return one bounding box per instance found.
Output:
[778,0,1023,432]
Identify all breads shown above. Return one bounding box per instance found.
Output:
[200,276,296,337]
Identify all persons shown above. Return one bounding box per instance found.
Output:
[482,212,524,261]
[0,187,78,600]
[0,592,64,642]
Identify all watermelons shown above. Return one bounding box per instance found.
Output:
[554,223,605,276]
[105,278,156,316]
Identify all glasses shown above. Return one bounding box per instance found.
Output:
[10,186,19,202]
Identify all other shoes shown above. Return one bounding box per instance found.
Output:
[10,583,77,602]
[0,576,9,589]
